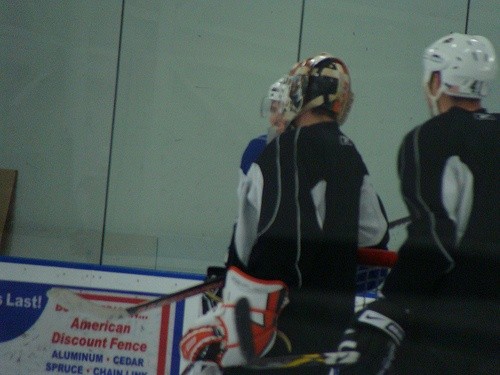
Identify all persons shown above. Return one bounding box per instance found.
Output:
[236,56,389,354]
[380,31,500,375]
[240,74,299,177]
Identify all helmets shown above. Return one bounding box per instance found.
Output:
[287,52,351,114]
[423,31,496,100]
[270,75,293,104]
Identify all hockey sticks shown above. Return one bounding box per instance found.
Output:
[235,298,361,368]
[47,275,225,321]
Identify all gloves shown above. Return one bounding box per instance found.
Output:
[339,295,407,375]
[186,264,287,370]
